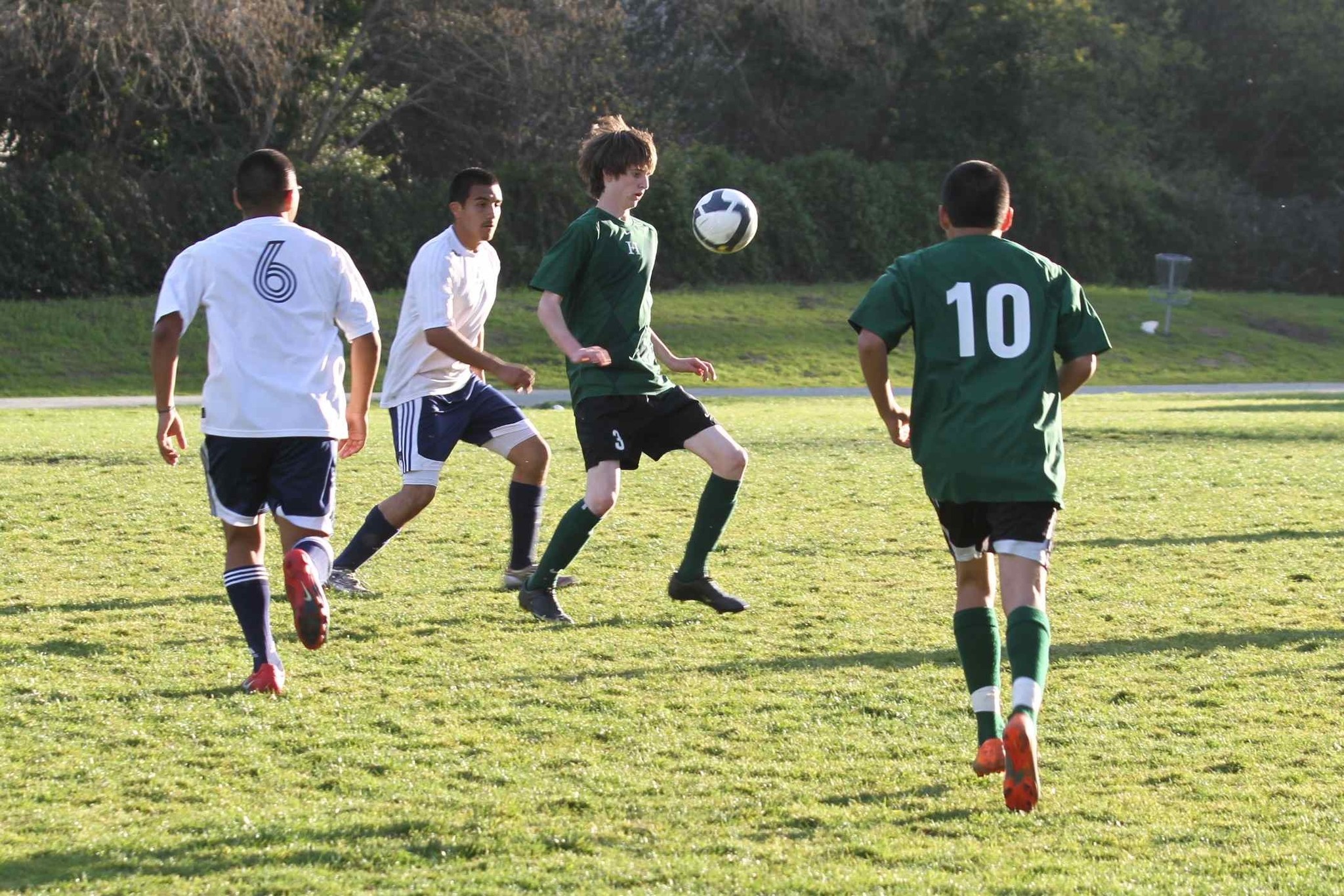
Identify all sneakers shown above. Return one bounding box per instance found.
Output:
[1002,714,1041,811]
[502,565,578,589]
[518,578,574,625]
[972,739,1007,778]
[667,571,749,615]
[322,568,373,595]
[283,549,329,649]
[241,663,286,694]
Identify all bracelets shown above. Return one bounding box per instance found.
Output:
[158,406,175,414]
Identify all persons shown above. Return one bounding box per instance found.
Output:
[850,159,1112,813]
[328,169,584,597]
[519,114,750,626]
[156,148,383,695]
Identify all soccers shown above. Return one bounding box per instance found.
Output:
[690,188,759,256]
